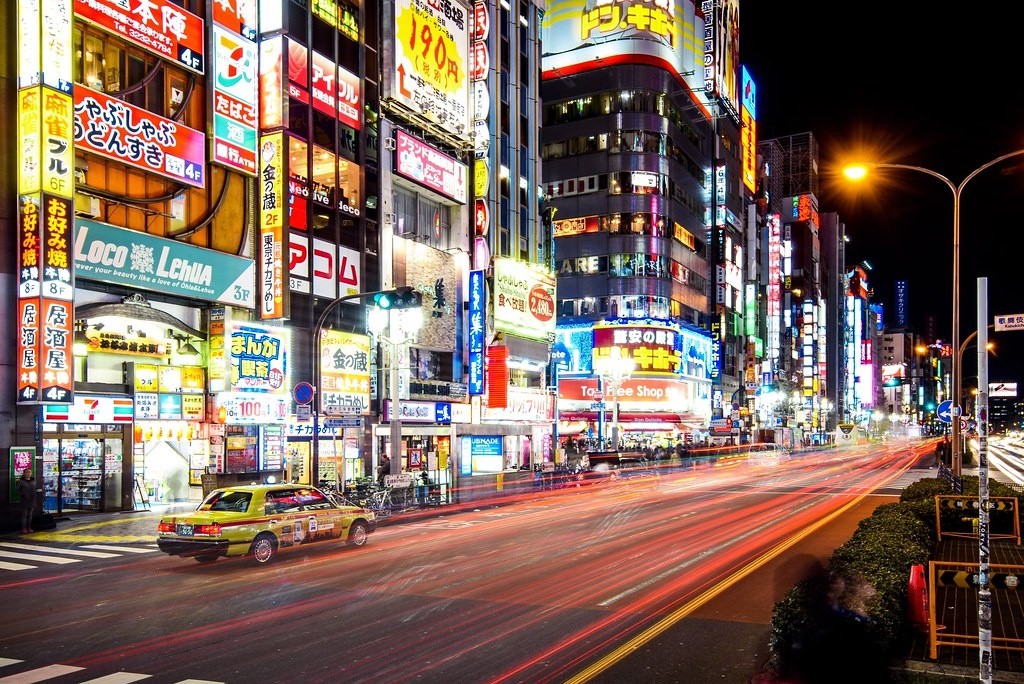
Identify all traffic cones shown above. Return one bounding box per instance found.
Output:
[906,564,946,634]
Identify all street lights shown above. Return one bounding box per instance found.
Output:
[592,346,637,450]
[837,148,1024,488]
[913,339,995,460]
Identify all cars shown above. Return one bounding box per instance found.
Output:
[156,482,377,565]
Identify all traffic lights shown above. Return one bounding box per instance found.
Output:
[371,287,423,311]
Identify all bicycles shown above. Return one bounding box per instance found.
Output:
[318,472,441,515]
[532,461,586,491]
